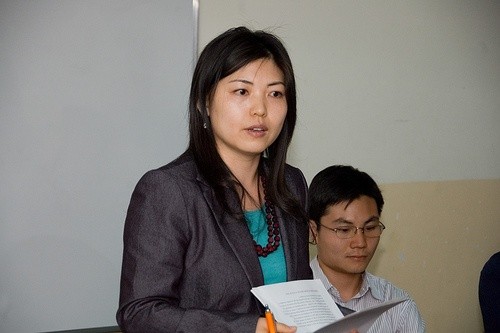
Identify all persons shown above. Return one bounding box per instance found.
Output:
[306,166,426,333]
[116,26,357,333]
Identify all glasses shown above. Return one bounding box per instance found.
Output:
[318,221,385,239]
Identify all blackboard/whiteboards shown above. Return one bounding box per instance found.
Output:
[0,1,201,332]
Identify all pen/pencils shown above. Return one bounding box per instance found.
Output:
[265,304,277,333]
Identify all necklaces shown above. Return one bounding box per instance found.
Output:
[244,163,280,256]
